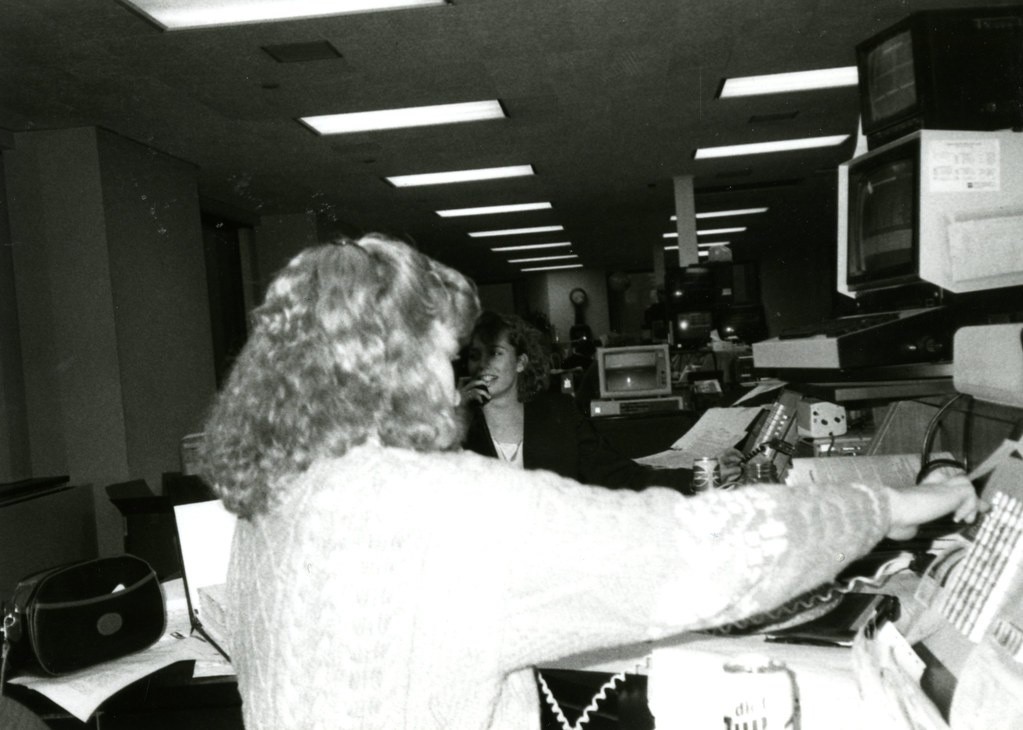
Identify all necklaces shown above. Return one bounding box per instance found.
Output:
[493,436,522,462]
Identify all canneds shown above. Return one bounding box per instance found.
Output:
[692,456,720,495]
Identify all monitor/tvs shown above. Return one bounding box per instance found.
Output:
[837,128,1023,307]
[597,343,673,399]
[855,8,1023,143]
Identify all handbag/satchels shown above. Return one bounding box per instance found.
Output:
[0,552,167,703]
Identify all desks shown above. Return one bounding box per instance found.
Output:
[796,380,964,468]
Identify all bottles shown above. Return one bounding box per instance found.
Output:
[740,461,778,486]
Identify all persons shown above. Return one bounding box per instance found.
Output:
[197,232,993,730]
[456,311,582,484]
